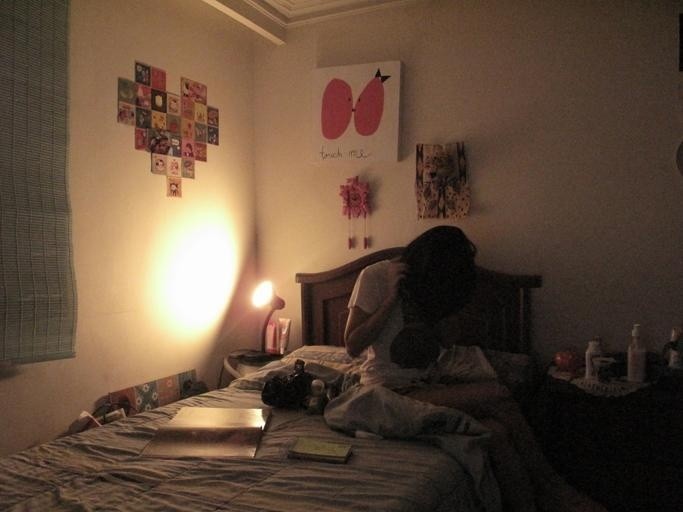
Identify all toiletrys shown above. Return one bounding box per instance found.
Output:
[627,323,647,382]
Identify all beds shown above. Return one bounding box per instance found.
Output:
[0,247,543,512]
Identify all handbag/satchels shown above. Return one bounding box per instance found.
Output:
[261,359,344,408]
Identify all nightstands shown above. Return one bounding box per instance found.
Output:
[534,360,683,512]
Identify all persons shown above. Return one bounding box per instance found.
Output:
[343,225,605,511]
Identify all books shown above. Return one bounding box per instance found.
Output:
[286,436,352,464]
[140,405,274,462]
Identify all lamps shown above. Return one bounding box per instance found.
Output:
[244,281,285,362]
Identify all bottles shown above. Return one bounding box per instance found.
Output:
[662,327,682,371]
